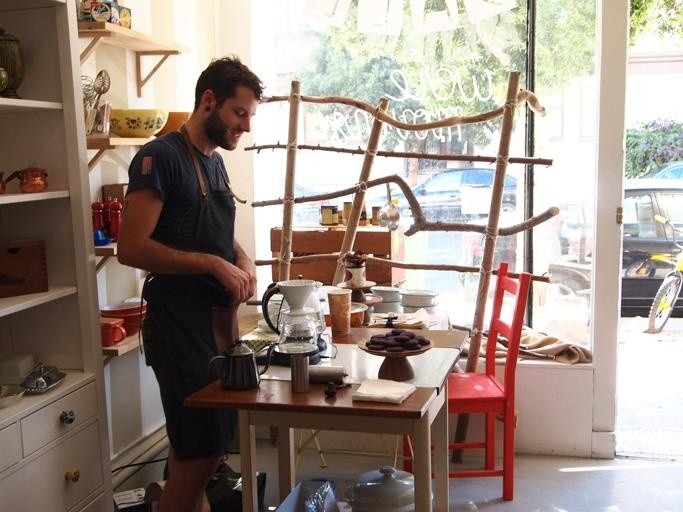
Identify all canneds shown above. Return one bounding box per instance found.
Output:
[319,202,380,226]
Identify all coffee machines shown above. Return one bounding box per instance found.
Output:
[258,280,328,351]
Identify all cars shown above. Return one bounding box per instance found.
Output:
[471,180,682,320]
[641,161,682,182]
[378,166,517,230]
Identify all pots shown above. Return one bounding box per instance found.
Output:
[344,462,416,511]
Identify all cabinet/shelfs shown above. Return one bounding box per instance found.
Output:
[78,20,180,367]
[0,0,112,512]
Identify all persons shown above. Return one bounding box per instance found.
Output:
[117,53,266,512]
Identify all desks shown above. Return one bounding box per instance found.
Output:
[182,325,469,512]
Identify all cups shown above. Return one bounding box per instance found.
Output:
[327,289,353,338]
[289,353,309,395]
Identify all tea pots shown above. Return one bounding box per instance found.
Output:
[210,337,281,392]
[0,169,19,195]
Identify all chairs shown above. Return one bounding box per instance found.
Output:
[403,261,531,500]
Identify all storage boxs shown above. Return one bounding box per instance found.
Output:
[0,237,48,298]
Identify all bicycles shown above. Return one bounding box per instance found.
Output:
[647,215,683,334]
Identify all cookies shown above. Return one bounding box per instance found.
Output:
[366,330,430,352]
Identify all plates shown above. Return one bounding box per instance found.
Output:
[19,372,66,394]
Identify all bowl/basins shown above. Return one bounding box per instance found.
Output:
[369,286,400,304]
[320,300,368,328]
[154,111,191,136]
[258,318,275,333]
[401,290,438,307]
[0,384,26,408]
[98,108,170,140]
[98,302,146,336]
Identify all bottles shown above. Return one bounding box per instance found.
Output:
[319,201,381,225]
[307,364,349,384]
[17,167,48,193]
[91,194,123,242]
